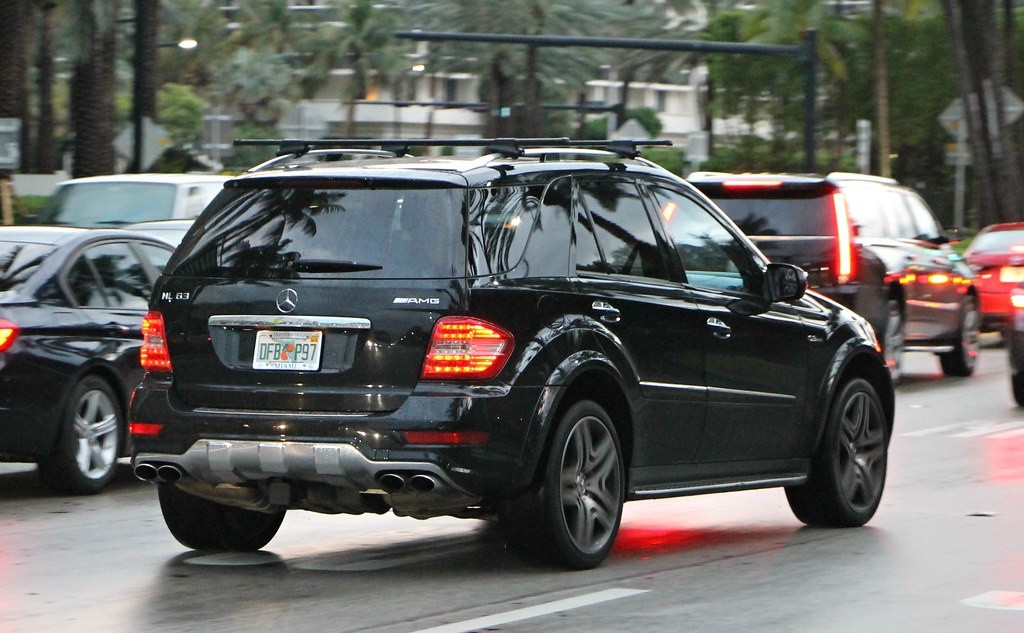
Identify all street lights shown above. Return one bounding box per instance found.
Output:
[393,64,426,139]
[580,62,612,140]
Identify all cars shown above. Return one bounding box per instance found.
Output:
[963,221,1024,408]
[0,224,176,496]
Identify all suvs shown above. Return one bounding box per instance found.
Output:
[126,135,895,571]
[37,171,232,247]
[663,168,981,387]
